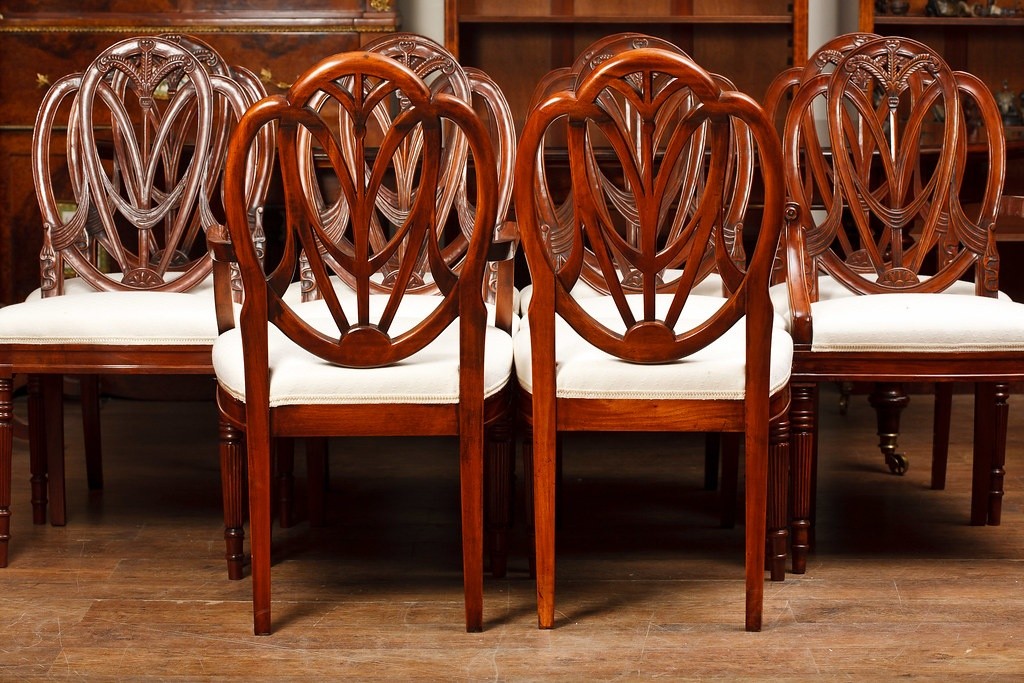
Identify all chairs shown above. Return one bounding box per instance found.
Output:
[1,33,1024,632]
